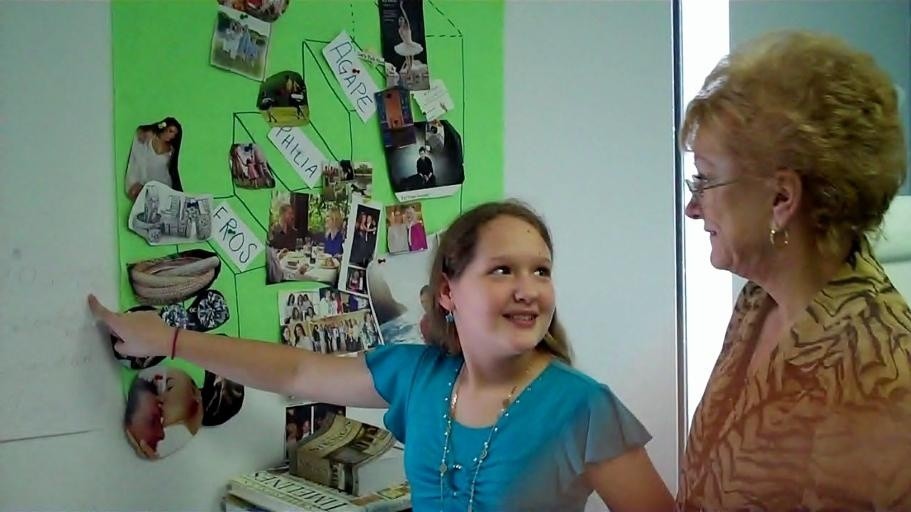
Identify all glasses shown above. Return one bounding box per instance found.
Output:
[686,175,749,202]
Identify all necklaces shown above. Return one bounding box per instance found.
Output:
[438,357,539,512]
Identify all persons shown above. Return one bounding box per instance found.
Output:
[416,147,436,187]
[127,367,198,459]
[223,14,257,67]
[286,420,311,458]
[85,199,674,512]
[127,116,184,199]
[641,31,911,511]
[268,202,432,353]
[284,74,304,120]
[259,93,278,124]
[231,145,274,189]
[393,0,427,83]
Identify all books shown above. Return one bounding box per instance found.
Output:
[224,411,411,512]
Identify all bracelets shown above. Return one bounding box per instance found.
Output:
[171,327,178,361]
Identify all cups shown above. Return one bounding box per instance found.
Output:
[287,257,299,268]
